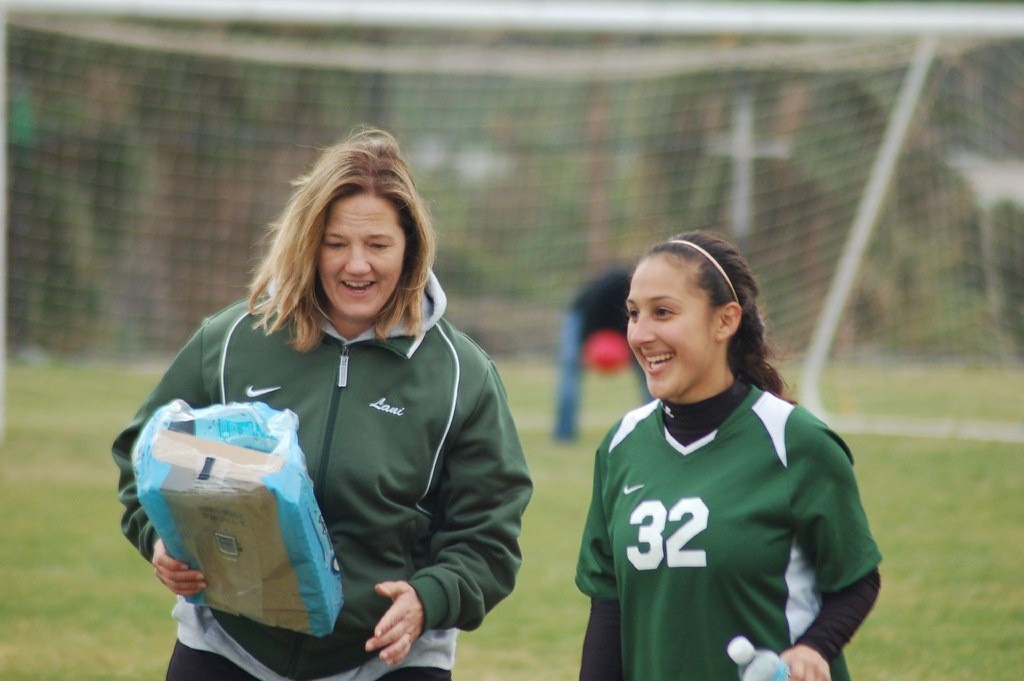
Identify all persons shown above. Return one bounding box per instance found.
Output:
[111,129,533,681]
[553,231,882,681]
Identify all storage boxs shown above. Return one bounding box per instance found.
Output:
[132,398,345,639]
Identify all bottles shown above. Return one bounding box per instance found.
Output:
[726,636,791,681]
